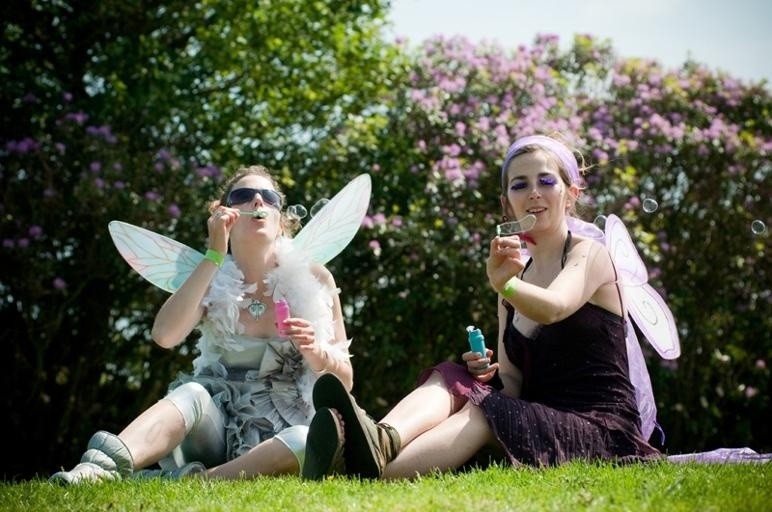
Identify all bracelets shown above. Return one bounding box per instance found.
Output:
[332,354,339,372]
[498,277,517,297]
[203,249,225,267]
[310,350,330,374]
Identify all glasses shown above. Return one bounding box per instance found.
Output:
[225,187,285,212]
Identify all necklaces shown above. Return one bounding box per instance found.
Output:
[245,291,269,323]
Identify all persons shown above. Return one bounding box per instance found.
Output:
[301,133,663,483]
[43,161,359,491]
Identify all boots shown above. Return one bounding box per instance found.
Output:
[309,373,400,482]
[131,462,206,484]
[300,409,345,484]
[45,429,135,492]
